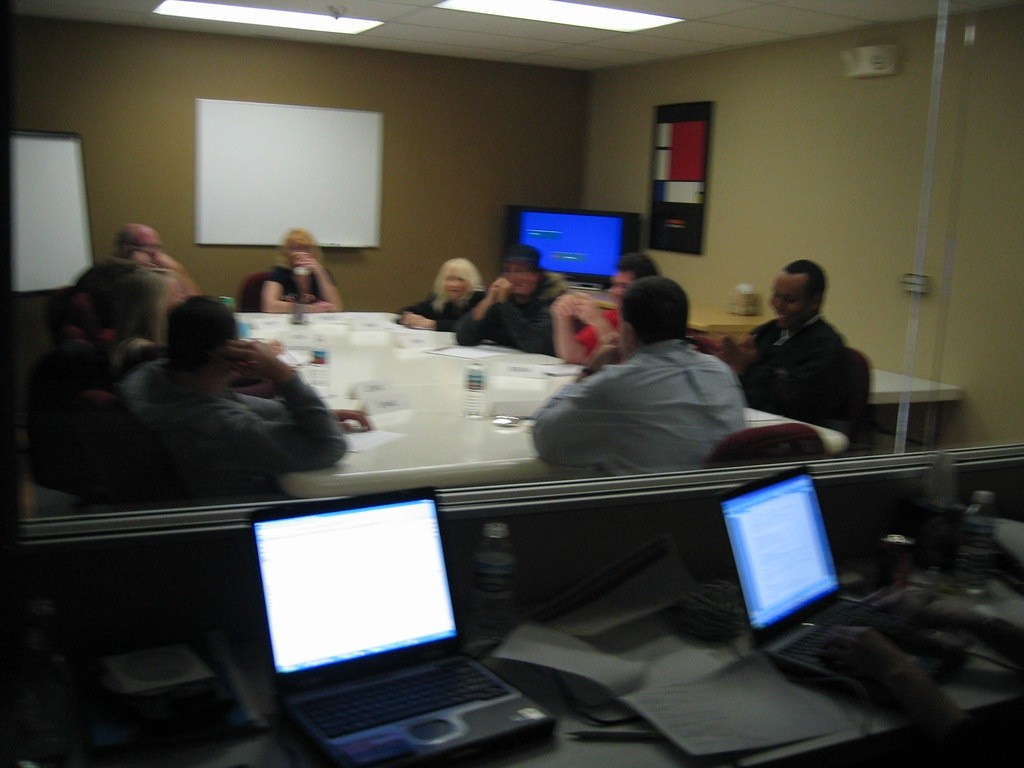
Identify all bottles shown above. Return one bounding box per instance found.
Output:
[959,491,996,596]
[311,341,328,388]
[472,523,517,636]
[467,361,488,420]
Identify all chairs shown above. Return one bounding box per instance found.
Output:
[845,348,868,441]
[706,423,827,466]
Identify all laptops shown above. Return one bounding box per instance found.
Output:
[712,464,978,708]
[251,487,558,768]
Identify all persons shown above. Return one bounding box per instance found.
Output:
[454,244,567,356]
[549,251,659,366]
[22,223,372,518]
[534,277,745,476]
[260,229,343,313]
[739,259,876,451]
[398,257,487,332]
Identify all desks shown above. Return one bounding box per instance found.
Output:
[686,307,774,345]
[1,555,1023,767]
[867,369,962,404]
[232,312,850,497]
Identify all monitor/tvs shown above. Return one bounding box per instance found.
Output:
[506,205,641,289]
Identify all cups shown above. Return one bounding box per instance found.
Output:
[293,305,303,324]
[290,251,311,276]
[881,535,915,588]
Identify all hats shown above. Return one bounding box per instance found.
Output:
[505,245,541,268]
[113,222,163,247]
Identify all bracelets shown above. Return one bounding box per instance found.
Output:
[583,368,595,375]
